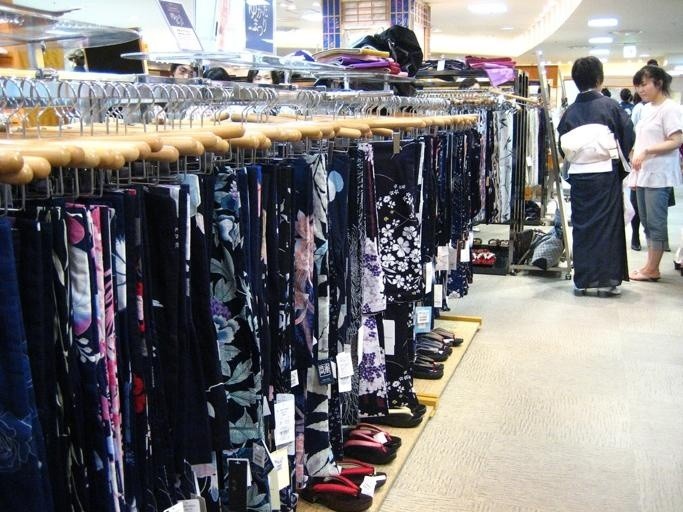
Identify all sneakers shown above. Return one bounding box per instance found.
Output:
[631,237,641,251]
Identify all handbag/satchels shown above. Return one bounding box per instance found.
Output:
[554,201,560,227]
[469,238,509,267]
[532,226,564,271]
[526,200,540,220]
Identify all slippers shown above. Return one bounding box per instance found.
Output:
[331,432,397,464]
[338,424,402,448]
[416,332,455,348]
[321,461,387,488]
[402,364,444,379]
[427,328,464,345]
[405,345,448,361]
[418,339,453,356]
[404,355,445,369]
[574,284,586,296]
[597,282,621,298]
[300,475,373,512]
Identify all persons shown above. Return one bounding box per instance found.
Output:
[66,48,86,73]
[619,88,634,117]
[460,79,481,90]
[171,64,197,83]
[633,92,641,105]
[626,58,683,282]
[601,87,611,98]
[556,55,636,298]
[646,59,672,86]
[248,70,280,86]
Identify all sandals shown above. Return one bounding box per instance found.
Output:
[629,270,662,281]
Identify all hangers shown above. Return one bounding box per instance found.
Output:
[0,76,406,210]
[407,87,522,133]
